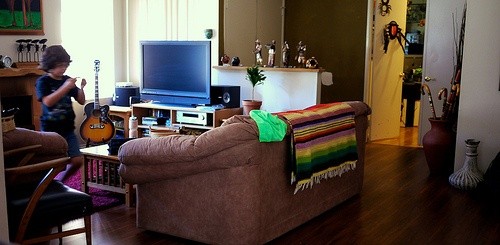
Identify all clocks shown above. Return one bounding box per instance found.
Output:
[3,56,12,68]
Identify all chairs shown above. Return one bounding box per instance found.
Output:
[5,145,92,245]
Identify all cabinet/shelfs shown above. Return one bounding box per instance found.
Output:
[131,103,244,138]
[108,105,131,138]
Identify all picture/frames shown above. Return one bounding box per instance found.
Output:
[0,0,45,35]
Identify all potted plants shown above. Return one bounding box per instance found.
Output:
[241,66,267,115]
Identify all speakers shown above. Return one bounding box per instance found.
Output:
[211,85,241,108]
[114,86,139,107]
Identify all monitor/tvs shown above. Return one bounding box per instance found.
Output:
[139,40,211,107]
[406,32,419,46]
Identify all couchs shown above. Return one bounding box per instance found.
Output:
[3,128,68,187]
[116,101,372,245]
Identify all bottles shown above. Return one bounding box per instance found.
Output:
[449,139,485,190]
[423,118,457,182]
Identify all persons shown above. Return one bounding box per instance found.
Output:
[306,57,319,69]
[294,41,306,68]
[252,39,263,66]
[35,45,87,158]
[282,40,294,68]
[265,39,276,68]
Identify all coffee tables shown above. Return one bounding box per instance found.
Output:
[80,143,134,207]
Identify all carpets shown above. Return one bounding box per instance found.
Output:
[64,155,122,212]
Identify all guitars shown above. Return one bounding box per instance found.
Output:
[78,59,116,146]
